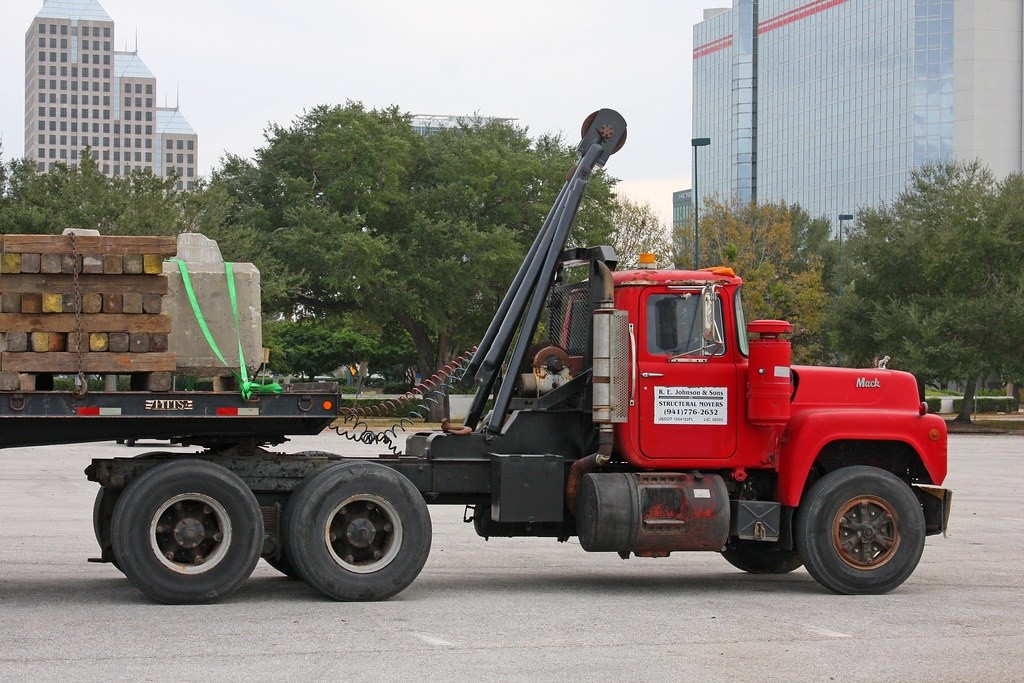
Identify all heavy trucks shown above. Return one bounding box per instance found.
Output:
[1,109,953,606]
